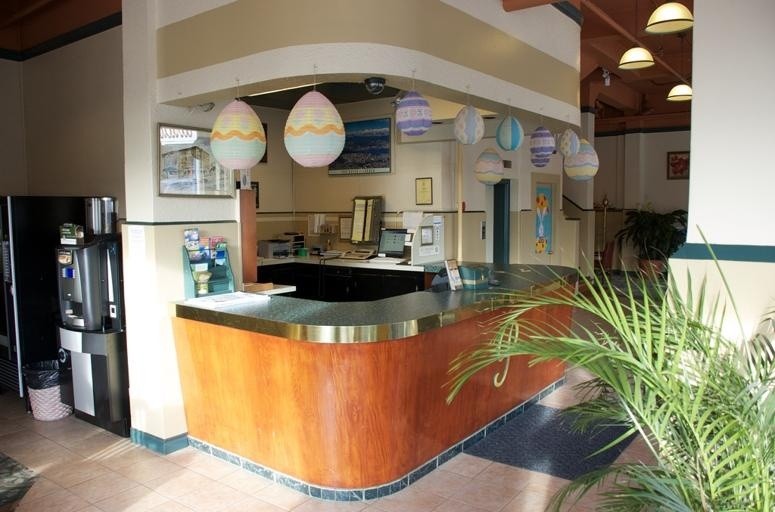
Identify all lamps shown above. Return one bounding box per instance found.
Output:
[618,0,655,70]
[645,0,694,34]
[666,32,693,102]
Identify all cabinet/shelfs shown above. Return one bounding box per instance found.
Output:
[182,238,236,299]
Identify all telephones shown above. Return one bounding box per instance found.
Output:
[339,248,377,261]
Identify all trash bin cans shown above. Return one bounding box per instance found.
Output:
[22,359,74,421]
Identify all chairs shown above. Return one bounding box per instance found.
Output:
[594,239,615,284]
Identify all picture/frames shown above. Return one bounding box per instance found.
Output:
[156,122,237,199]
[415,177,433,206]
[236,181,259,209]
[259,123,268,164]
[327,114,395,177]
[666,151,690,180]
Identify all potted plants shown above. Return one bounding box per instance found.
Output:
[614,208,688,280]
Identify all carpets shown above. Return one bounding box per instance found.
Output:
[463,403,644,485]
